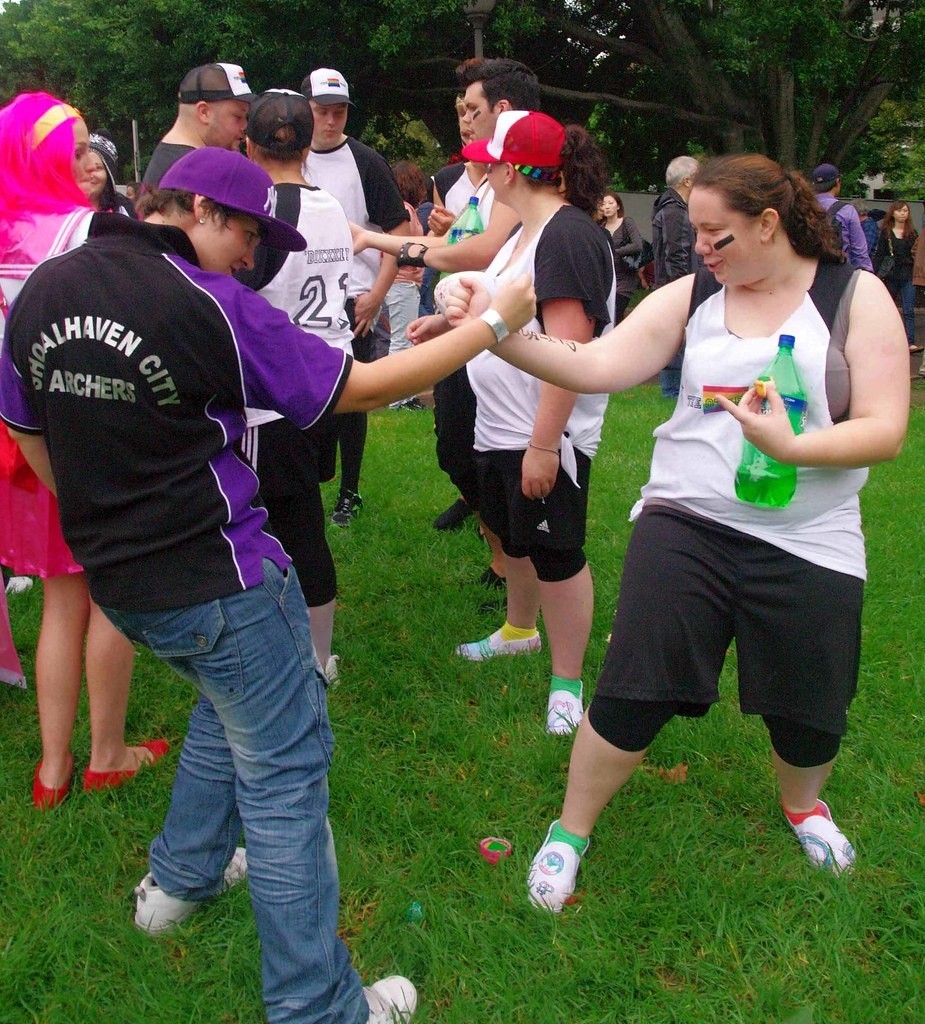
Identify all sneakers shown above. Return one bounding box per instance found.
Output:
[546,674,583,736]
[432,498,487,545]
[330,486,363,529]
[135,847,248,936]
[528,820,589,913]
[362,976,418,1024]
[456,627,542,661]
[4,577,34,596]
[784,799,856,879]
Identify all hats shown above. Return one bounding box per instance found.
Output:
[247,88,314,150]
[301,68,357,109]
[813,164,842,184]
[88,133,117,192]
[159,146,306,252]
[180,63,259,103]
[461,110,567,166]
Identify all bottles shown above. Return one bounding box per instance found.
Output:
[440,195,484,281]
[734,334,809,510]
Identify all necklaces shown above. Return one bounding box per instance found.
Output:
[768,290,774,294]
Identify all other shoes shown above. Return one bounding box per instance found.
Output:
[312,643,340,690]
[909,345,925,354]
[461,567,507,617]
[398,398,425,412]
[33,738,169,812]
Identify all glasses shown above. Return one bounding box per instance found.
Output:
[486,162,506,173]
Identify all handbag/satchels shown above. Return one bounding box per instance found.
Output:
[618,217,654,273]
[878,228,894,278]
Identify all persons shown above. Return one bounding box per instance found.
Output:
[82,133,142,221]
[127,182,142,202]
[445,152,911,911]
[139,59,925,692]
[408,110,617,734]
[0,147,535,1024]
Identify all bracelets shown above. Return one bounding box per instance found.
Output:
[479,308,510,343]
[528,441,559,454]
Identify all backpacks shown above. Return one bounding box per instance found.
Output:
[827,200,849,252]
